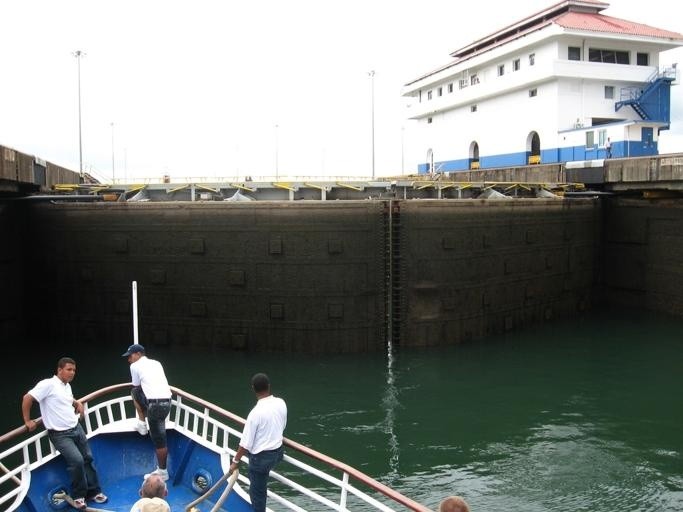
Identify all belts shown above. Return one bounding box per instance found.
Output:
[149,399,172,403]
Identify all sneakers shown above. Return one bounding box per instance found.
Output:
[144,468,169,481]
[91,493,108,504]
[73,498,87,508]
[133,419,148,435]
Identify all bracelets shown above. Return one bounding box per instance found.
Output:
[231,457,240,464]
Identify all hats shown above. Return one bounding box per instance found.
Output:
[121,345,144,357]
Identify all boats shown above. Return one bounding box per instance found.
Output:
[0,282,435,512]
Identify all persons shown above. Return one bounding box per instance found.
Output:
[121,344,173,482]
[129,474,171,512]
[439,495,470,512]
[229,372,288,511]
[605,137,612,158]
[22,358,108,509]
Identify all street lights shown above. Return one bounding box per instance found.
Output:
[367,69,379,180]
[72,48,87,177]
